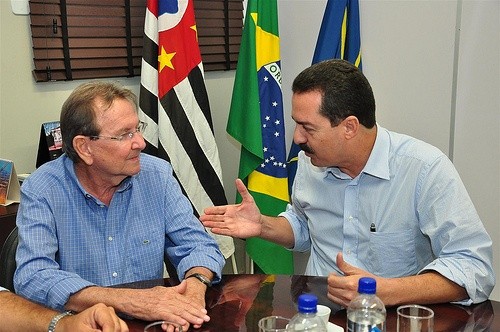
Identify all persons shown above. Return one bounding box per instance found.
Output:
[13,80,227,332]
[217,274,494,332]
[199,60,495,308]
[0,284,129,332]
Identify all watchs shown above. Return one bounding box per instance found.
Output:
[185,273,211,296]
[48,310,78,332]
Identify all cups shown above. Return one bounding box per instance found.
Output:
[396,304,434,332]
[258,316,291,332]
[144,321,183,332]
[316,304,331,327]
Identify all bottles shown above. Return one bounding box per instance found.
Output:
[346,277,387,332]
[287,293,328,332]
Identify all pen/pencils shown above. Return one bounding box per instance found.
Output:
[370,223,376,232]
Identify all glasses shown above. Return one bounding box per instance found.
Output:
[83,120,148,145]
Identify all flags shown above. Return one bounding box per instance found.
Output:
[286,0,362,207]
[138,0,234,278]
[226,0,294,274]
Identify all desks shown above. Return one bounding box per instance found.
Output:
[106,273,499,332]
